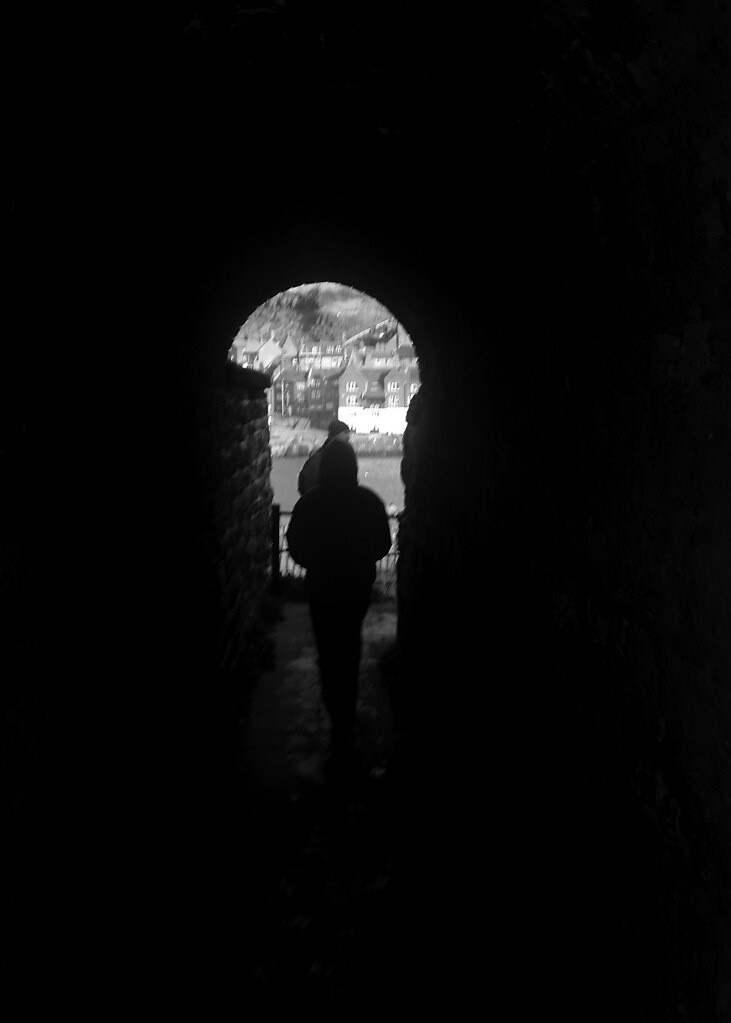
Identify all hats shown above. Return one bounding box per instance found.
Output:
[328,419,348,437]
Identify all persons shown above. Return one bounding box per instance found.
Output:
[286,420,391,751]
